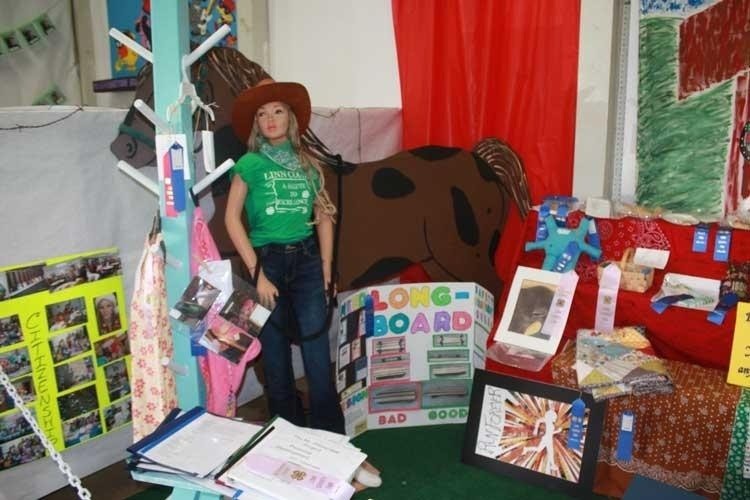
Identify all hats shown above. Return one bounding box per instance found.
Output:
[94,293,116,309]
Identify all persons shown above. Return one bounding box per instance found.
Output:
[1,350,28,376]
[521,408,563,473]
[223,78,347,438]
[49,303,83,328]
[55,332,90,359]
[230,293,256,332]
[106,399,132,428]
[1,434,44,469]
[95,295,119,332]
[0,316,21,346]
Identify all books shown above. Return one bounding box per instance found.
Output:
[369,334,470,404]
[124,406,372,500]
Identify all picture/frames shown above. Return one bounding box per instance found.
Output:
[461,368,608,500]
[492,263,580,356]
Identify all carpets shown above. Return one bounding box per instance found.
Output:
[125,409,748,500]
